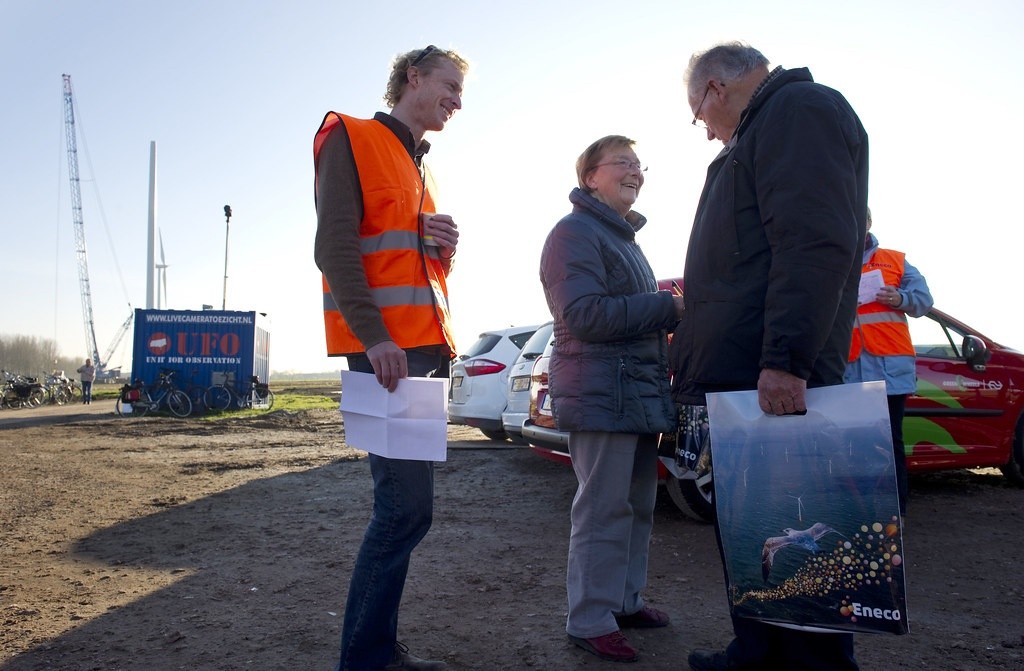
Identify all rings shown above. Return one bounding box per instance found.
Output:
[890,298,892,300]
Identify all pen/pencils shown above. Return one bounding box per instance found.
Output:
[671,280,683,296]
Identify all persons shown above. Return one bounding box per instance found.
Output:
[77,359,96,405]
[837,208,933,528]
[666,46,868,671]
[313,47,467,671]
[540,136,683,660]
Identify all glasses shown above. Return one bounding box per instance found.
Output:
[409,45,438,65]
[692,79,725,129]
[588,158,649,175]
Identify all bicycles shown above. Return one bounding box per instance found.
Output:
[0,368,83,410]
[203,368,274,412]
[116,368,192,417]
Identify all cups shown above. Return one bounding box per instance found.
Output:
[422,211,448,247]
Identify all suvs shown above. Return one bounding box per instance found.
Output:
[446,323,541,441]
[518,280,1024,525]
[500,319,555,443]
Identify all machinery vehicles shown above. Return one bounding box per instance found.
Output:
[59,71,135,384]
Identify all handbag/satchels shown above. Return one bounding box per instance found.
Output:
[704,381,909,637]
[254,382,268,398]
[121,390,140,403]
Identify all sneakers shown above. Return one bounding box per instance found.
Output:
[687,649,736,671]
[611,607,670,629]
[383,639,448,671]
[567,629,641,663]
